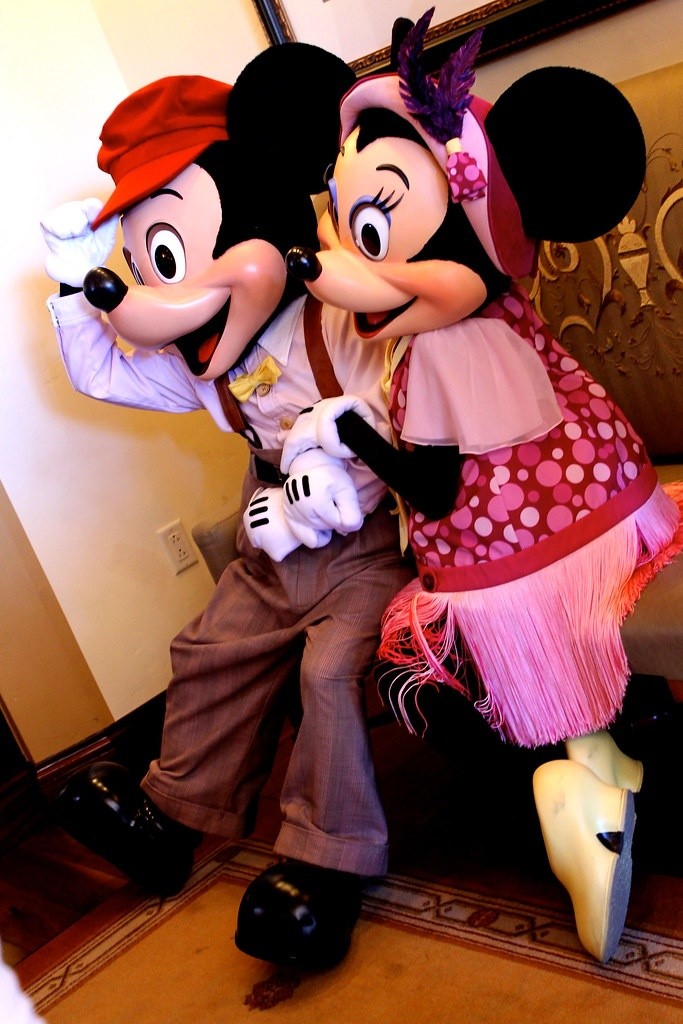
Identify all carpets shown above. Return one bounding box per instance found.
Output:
[9,833,683,1024]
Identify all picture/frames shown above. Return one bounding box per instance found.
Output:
[250,0,662,81]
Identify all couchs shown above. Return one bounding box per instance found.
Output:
[191,60,683,736]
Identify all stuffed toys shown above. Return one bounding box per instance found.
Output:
[278,5,683,963]
[39,44,416,970]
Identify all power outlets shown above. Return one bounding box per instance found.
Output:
[156,518,198,577]
